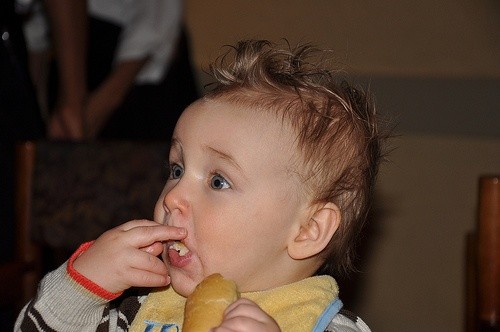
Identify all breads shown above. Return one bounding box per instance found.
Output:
[181,272,241,332]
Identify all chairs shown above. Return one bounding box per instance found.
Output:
[17,140,171,306]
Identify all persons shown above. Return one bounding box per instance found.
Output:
[0,0,87,314]
[13,39,399,332]
[19,0,201,144]
